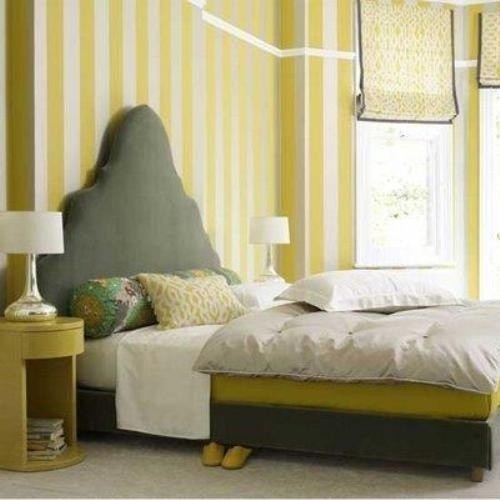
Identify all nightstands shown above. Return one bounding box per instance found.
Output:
[0,317,90,473]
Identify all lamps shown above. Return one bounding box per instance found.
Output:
[247,216,291,283]
[0,211,65,321]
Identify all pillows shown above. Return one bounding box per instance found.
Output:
[70,266,240,339]
[138,274,246,328]
[272,266,469,313]
[229,282,292,309]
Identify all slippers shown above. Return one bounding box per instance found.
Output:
[202,442,252,469]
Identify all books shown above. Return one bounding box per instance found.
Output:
[27,414,68,462]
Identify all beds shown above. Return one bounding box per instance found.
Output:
[37,105,499,482]
